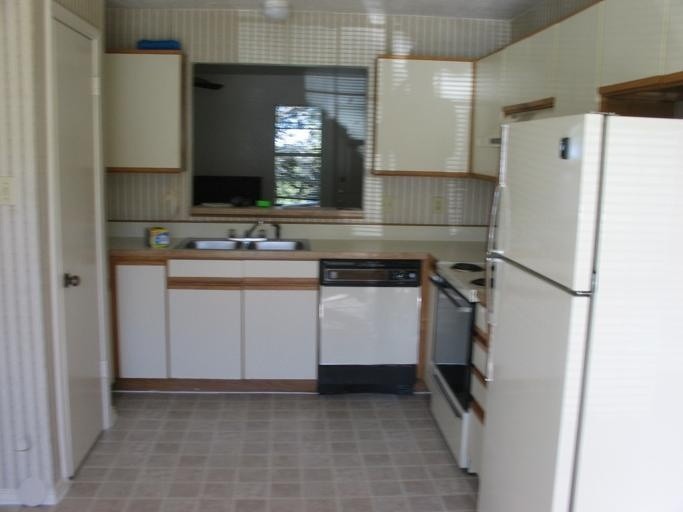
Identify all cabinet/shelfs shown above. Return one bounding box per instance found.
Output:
[109,255,167,390]
[467,292,488,476]
[167,258,320,392]
[102,50,186,173]
[372,54,474,178]
[471,1,683,181]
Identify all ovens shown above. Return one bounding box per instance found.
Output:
[425,273,476,469]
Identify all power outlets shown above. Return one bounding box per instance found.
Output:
[432,197,444,214]
[379,196,394,212]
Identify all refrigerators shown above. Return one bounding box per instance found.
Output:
[478,116,682,512]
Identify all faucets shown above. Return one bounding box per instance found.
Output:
[243,218,263,237]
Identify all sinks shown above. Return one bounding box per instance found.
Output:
[175,236,242,250]
[244,240,309,250]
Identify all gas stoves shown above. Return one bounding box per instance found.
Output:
[321,259,421,390]
